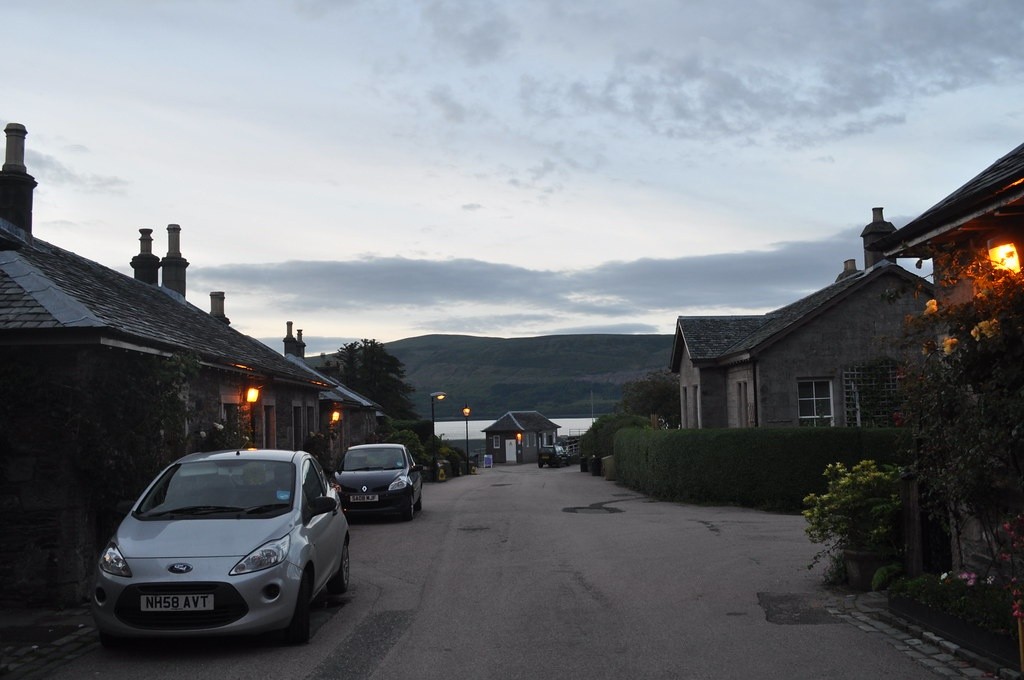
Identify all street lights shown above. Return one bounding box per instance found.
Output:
[462,403,471,475]
[429,391,448,462]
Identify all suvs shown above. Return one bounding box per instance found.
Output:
[538,444,571,469]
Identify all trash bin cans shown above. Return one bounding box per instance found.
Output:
[469,453,479,468]
[460,461,474,474]
[601,455,617,480]
[589,458,601,476]
[579,457,587,471]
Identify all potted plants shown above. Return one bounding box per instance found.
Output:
[799,457,902,592]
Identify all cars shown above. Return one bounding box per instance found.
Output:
[328,443,424,522]
[91,447,351,647]
